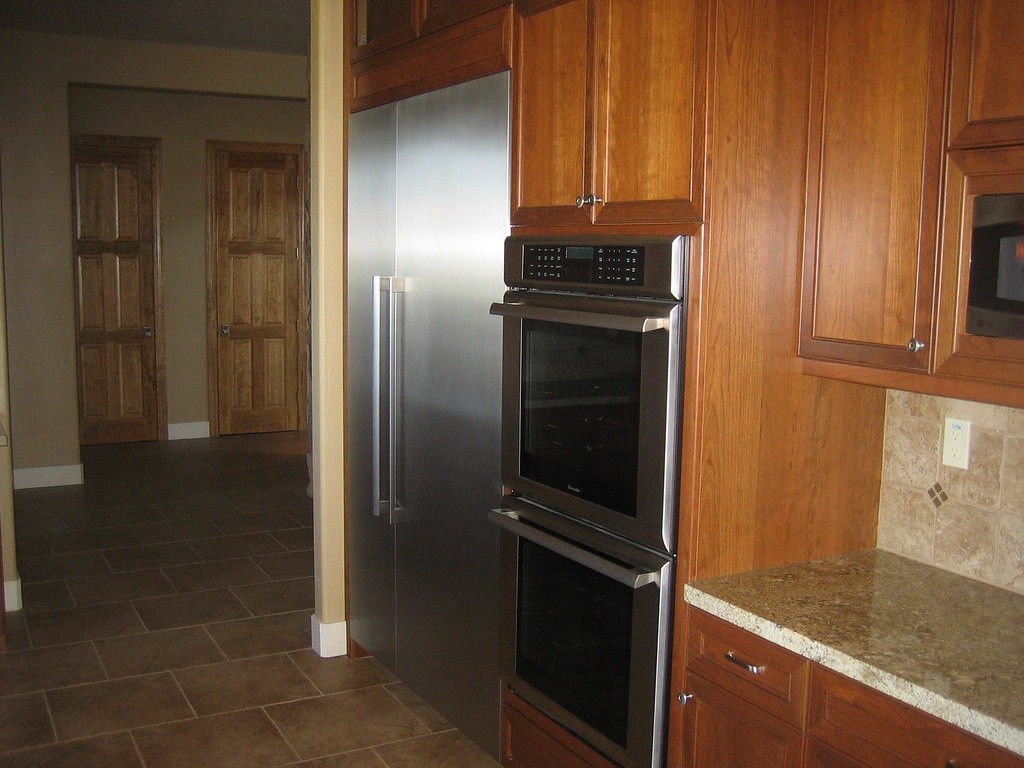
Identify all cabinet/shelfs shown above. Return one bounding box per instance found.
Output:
[682,548,1024,768]
[343,0,1024,410]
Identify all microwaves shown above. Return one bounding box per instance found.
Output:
[966,193,1024,339]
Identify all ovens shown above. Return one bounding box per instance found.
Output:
[489,235,690,768]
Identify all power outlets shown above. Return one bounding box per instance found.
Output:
[942,417,972,470]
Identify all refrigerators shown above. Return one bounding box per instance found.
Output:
[346,70,513,764]
